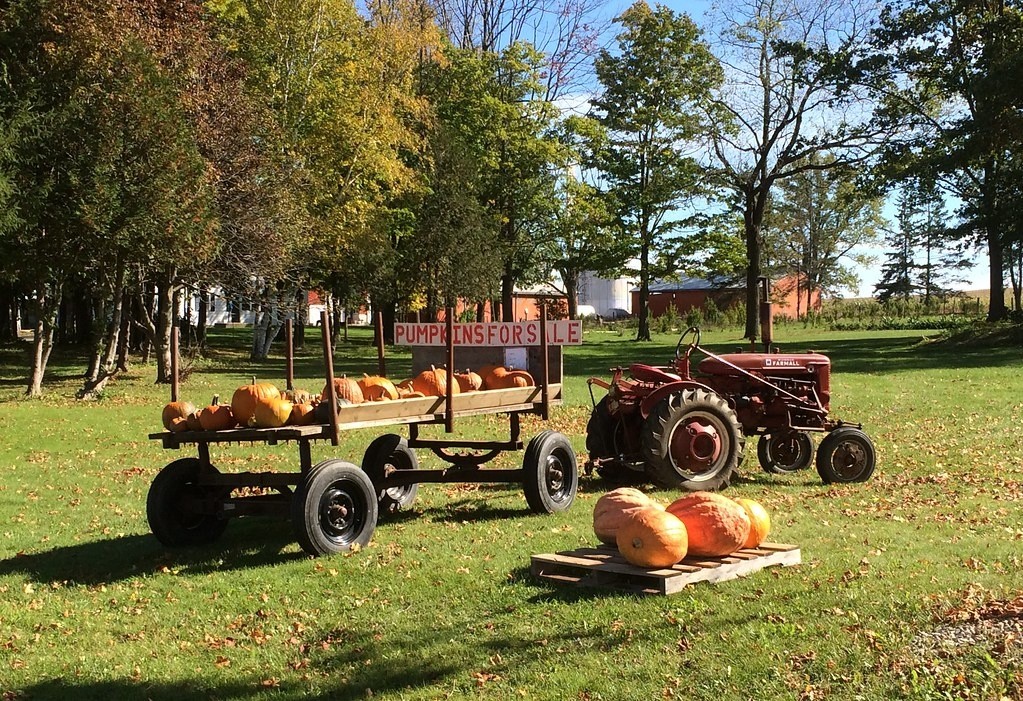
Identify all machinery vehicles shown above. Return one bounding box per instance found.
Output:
[583,329,876,492]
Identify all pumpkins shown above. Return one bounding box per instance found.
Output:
[162,362,536,432]
[592,487,770,568]
